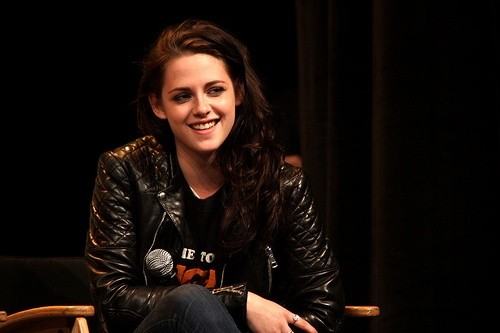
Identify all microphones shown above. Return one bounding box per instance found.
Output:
[145,248,181,287]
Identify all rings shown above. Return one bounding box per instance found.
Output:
[289,330,293,333]
[292,314,299,325]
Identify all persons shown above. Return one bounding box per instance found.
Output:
[85,19,348,333]
